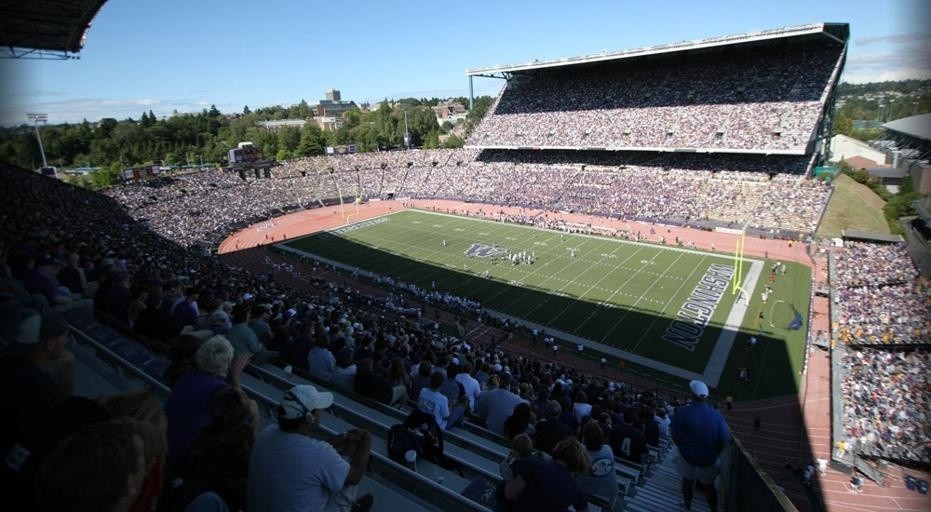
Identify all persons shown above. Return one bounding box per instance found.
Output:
[0,41,931,512]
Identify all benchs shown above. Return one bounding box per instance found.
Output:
[64,320,644,511]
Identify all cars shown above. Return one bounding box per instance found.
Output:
[904,475,928,495]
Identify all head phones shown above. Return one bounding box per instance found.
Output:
[282,389,316,424]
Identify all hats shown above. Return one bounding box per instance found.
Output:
[688,379,708,401]
[11,314,69,343]
[36,254,62,266]
[276,384,334,419]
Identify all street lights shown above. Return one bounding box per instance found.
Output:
[26,113,49,168]
